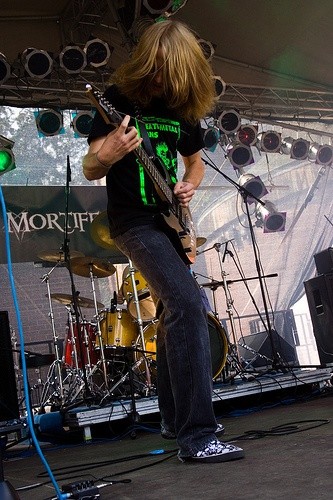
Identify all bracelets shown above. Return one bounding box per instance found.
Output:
[95,154,109,169]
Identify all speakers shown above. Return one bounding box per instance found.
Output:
[233,329,301,370]
[303,274,333,367]
[0,311,20,422]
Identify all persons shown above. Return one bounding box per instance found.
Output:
[83,19,244,465]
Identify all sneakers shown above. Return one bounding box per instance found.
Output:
[178,438,245,463]
[160,423,225,439]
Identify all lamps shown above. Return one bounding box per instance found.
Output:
[142,0,175,15]
[70,104,94,137]
[83,37,115,68]
[0,53,14,84]
[224,140,254,170]
[281,136,310,161]
[196,38,217,63]
[238,173,268,205]
[236,124,258,147]
[212,107,242,135]
[35,99,63,136]
[58,38,87,74]
[256,200,287,234]
[256,130,281,153]
[18,47,53,79]
[201,128,217,151]
[0,135,17,176]
[309,142,333,165]
[212,75,226,101]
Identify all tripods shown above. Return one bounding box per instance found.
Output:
[25,259,159,416]
[195,238,273,381]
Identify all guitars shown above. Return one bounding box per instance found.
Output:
[81,84,196,265]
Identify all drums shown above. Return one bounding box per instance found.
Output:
[122,264,158,322]
[94,308,139,355]
[62,319,100,372]
[134,313,230,385]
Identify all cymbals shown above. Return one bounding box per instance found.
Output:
[196,237,208,249]
[66,257,116,278]
[91,211,119,251]
[44,292,106,309]
[37,249,85,263]
